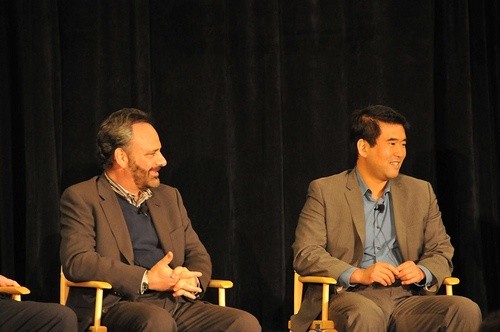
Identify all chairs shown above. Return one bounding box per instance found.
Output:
[60,265,234,332]
[288,269,459,332]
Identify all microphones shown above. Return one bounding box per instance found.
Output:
[137,208,144,215]
[377,204,385,213]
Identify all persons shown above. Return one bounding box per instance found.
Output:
[58,107,263,332]
[0,275,79,332]
[289,104,483,332]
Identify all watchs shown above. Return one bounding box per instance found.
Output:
[141,282,149,293]
